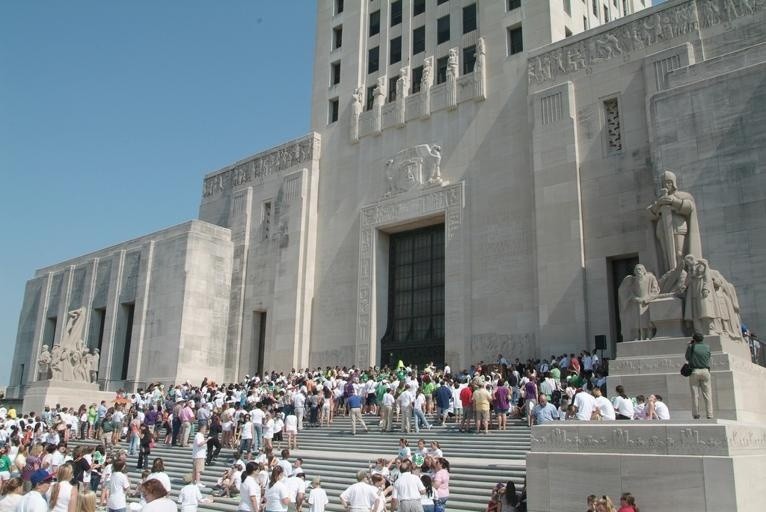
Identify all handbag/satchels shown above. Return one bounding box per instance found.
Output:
[680,364,693,377]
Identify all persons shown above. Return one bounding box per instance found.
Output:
[751,332,759,362]
[739,321,748,339]
[202,137,313,201]
[681,332,716,418]
[1,349,669,512]
[352,37,486,116]
[525,1,766,93]
[618,171,742,341]
[36,307,101,382]
